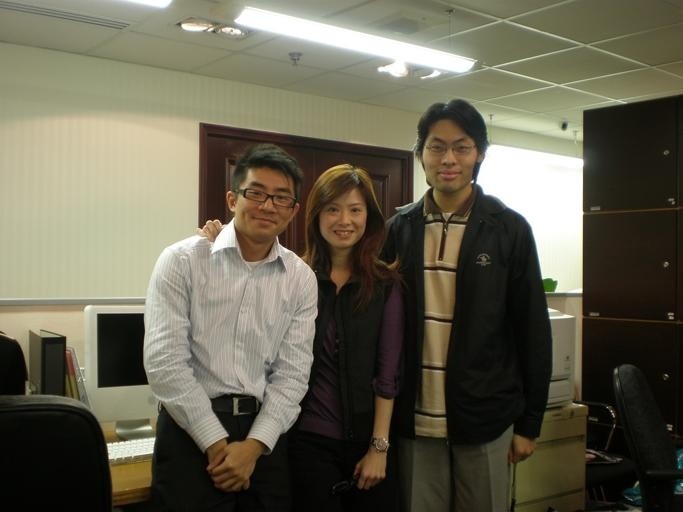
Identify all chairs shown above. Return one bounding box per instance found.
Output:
[573,363,683,512]
[0,395,115,512]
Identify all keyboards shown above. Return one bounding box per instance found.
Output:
[106,437,157,465]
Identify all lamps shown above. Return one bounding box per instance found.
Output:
[233,0,480,74]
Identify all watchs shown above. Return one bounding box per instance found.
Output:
[369,435,390,454]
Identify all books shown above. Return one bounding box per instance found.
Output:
[64,347,91,409]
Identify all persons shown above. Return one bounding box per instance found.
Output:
[192,162,408,510]
[142,142,320,512]
[374,97,553,510]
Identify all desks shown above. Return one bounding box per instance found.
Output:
[97,418,161,509]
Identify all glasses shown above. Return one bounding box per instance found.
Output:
[234,188,297,208]
[428,143,476,155]
[332,473,359,493]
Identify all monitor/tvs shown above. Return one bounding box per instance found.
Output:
[83,305,160,441]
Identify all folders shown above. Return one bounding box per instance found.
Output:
[28,329,66,396]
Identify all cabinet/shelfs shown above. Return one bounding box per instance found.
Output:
[506,405,588,512]
[580,94,682,467]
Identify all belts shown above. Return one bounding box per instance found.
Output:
[211,395,260,413]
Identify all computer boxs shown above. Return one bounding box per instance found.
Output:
[545,308,577,410]
